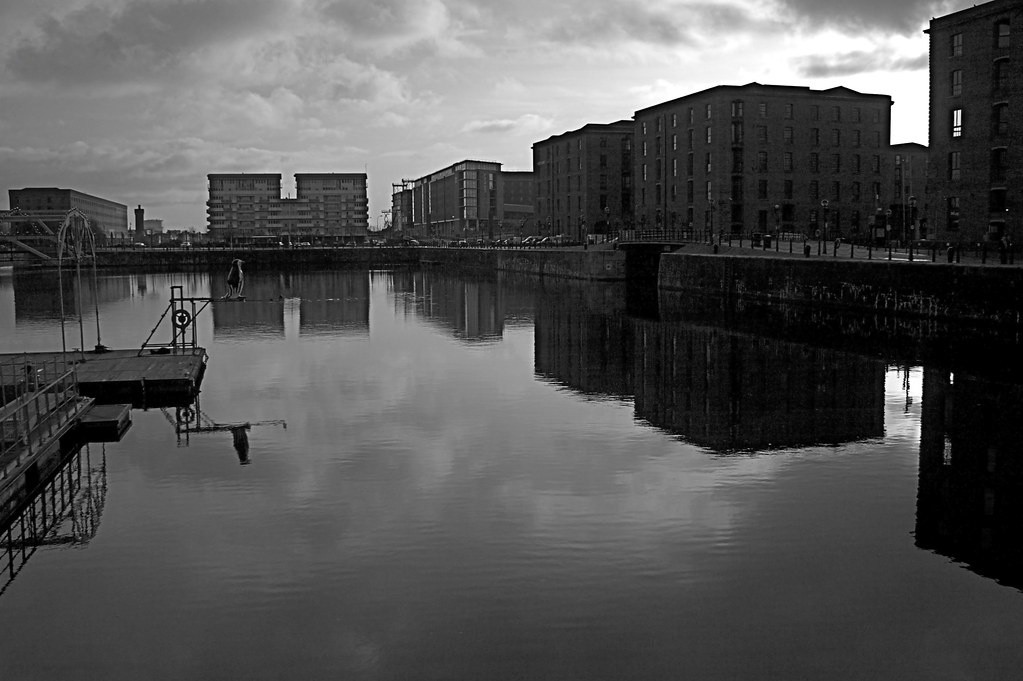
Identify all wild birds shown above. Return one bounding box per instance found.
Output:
[221,259,245,298]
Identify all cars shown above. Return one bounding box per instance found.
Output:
[135,242,144,247]
[272,241,310,247]
[346,241,357,247]
[180,241,191,248]
[448,234,619,247]
[407,239,419,246]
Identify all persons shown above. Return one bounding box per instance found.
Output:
[998,235,1009,265]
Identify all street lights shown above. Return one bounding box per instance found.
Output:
[500,224,502,248]
[774,205,780,252]
[480,223,485,247]
[548,222,550,247]
[821,199,828,255]
[907,196,916,261]
[709,198,716,245]
[520,224,524,248]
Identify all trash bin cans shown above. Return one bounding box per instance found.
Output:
[753,233,761,246]
[764,235,772,248]
